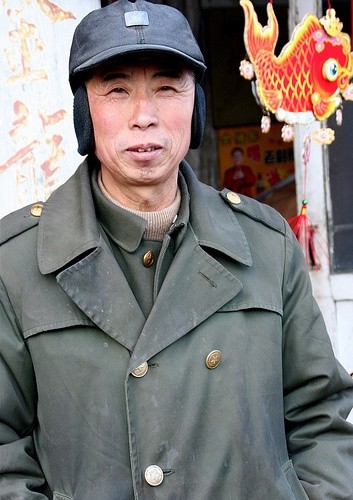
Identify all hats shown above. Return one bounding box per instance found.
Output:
[68,0,207,97]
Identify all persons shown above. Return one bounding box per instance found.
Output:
[1,1,351,500]
[223,148,255,198]
